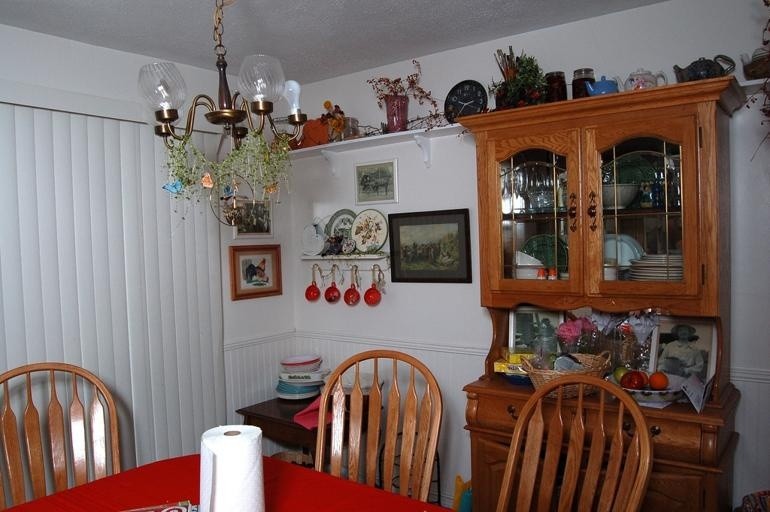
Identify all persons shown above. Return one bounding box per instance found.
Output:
[659,323,703,379]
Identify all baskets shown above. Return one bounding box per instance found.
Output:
[519,349,613,399]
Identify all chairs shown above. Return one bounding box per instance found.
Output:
[494,373,654,511]
[0,362,122,509]
[314,349,442,506]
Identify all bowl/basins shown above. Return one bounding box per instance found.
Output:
[281,355,323,373]
[603,183,639,210]
[528,190,554,208]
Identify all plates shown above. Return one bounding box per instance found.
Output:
[350,209,388,253]
[303,233,324,256]
[342,239,356,253]
[626,253,685,280]
[327,209,356,237]
[604,233,642,270]
[607,374,687,402]
[522,234,569,280]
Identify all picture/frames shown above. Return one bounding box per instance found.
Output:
[227,243,283,301]
[649,315,717,384]
[388,208,473,285]
[232,197,274,240]
[508,305,565,356]
[353,157,399,206]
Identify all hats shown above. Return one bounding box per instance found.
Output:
[671,323,696,335]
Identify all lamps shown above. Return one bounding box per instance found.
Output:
[134,43,307,149]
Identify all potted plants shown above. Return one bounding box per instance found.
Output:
[506,55,546,107]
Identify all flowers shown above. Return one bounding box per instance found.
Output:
[320,100,343,130]
[366,60,440,133]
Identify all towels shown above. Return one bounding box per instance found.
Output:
[293,394,332,430]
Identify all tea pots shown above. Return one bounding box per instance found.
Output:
[674,54,736,83]
[612,68,668,92]
[585,76,619,96]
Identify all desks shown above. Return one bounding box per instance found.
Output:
[235,393,384,471]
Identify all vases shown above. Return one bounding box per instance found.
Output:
[383,95,409,132]
[339,117,360,140]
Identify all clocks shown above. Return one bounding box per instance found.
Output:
[444,79,488,124]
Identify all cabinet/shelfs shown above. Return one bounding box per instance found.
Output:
[456,74,740,512]
[282,77,769,174]
